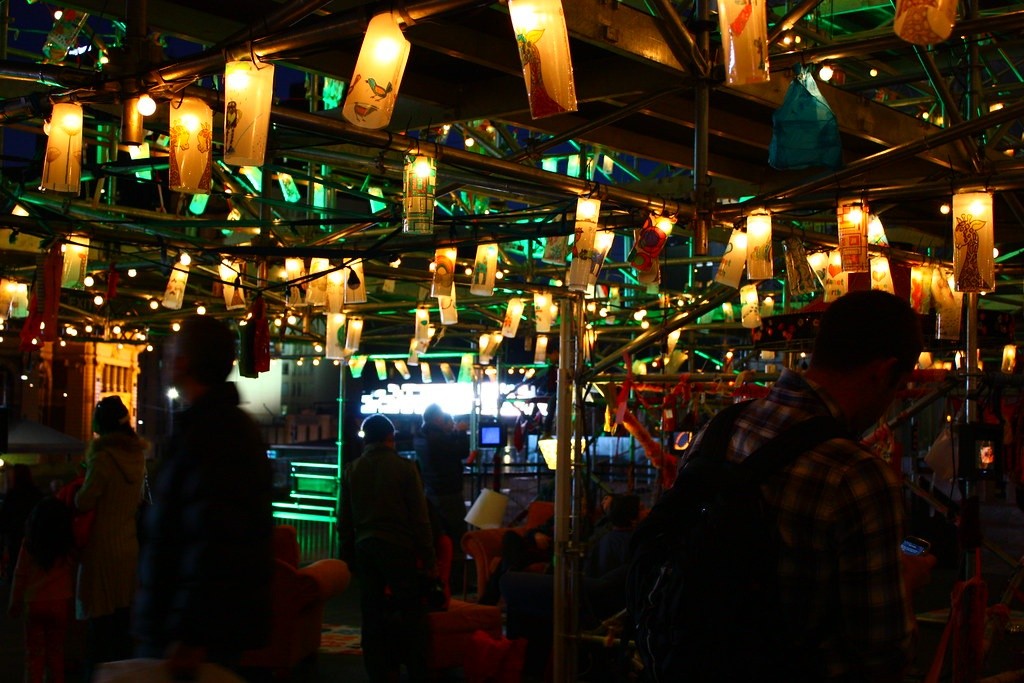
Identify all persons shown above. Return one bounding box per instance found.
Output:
[6,395,151,683]
[124,314,275,683]
[493,477,645,683]
[671,290,937,683]
[335,408,468,683]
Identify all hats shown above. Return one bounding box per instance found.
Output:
[95,397,130,428]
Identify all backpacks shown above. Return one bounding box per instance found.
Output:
[624,398,858,683]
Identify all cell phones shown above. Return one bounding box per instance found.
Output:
[900,536,929,556]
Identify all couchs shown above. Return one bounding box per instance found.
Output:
[239,499,651,683]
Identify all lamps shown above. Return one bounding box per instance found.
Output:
[37,0,1024,383]
[464,487,508,528]
